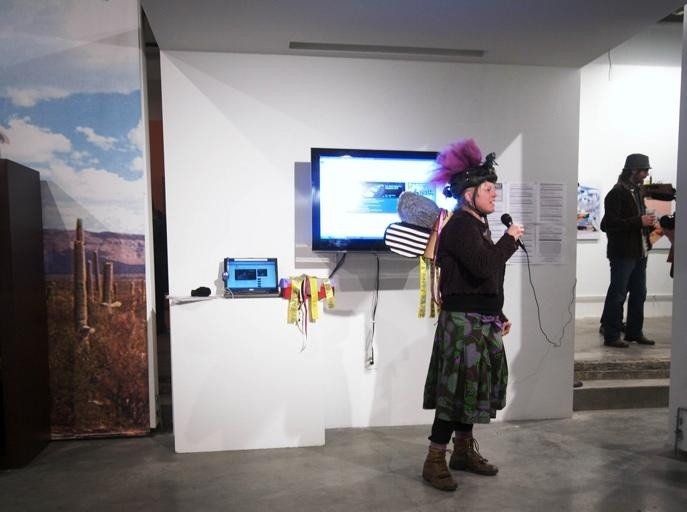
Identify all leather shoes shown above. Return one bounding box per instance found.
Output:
[623,331,657,345]
[603,337,629,348]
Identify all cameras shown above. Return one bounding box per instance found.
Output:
[660,215,675,229]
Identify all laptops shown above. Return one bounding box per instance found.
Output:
[223,257,282,298]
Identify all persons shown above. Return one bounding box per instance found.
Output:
[602,153,663,348]
[420,162,525,492]
[597,216,629,335]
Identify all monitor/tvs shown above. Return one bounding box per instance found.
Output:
[311,148,458,254]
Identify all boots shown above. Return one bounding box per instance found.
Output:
[423,448,458,491]
[449,436,499,476]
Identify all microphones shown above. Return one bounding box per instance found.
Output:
[501,214,528,254]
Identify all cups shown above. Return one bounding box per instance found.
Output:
[644,207,656,226]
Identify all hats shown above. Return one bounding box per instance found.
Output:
[447,164,498,199]
[621,153,653,170]
